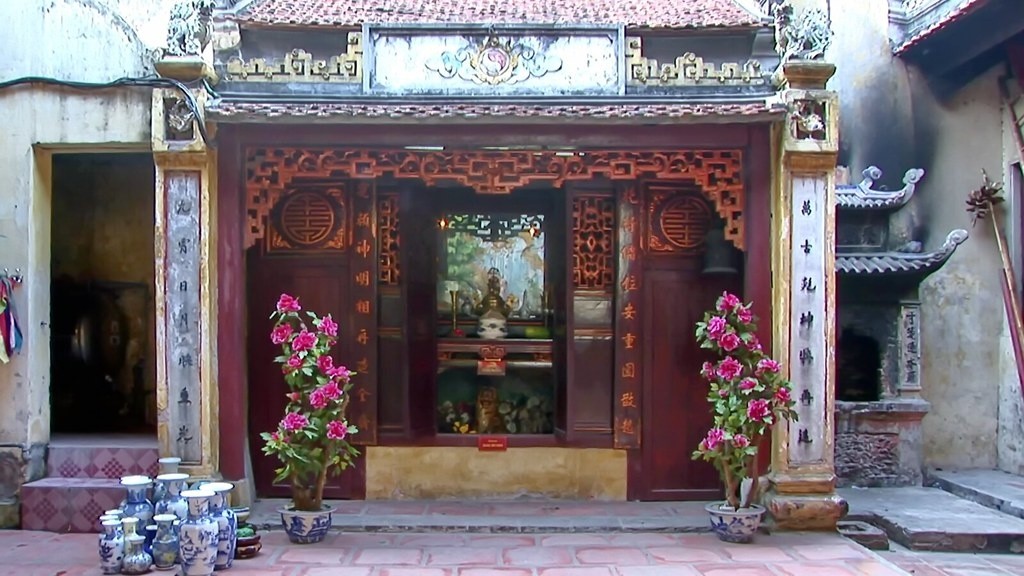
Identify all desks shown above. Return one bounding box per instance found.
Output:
[433,338,552,371]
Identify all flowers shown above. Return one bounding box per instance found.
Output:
[258,293,360,508]
[691,290,801,507]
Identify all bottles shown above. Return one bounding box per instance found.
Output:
[98,457,264,576]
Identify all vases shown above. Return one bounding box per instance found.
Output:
[276,504,334,544]
[96,455,261,576]
[706,503,767,546]
